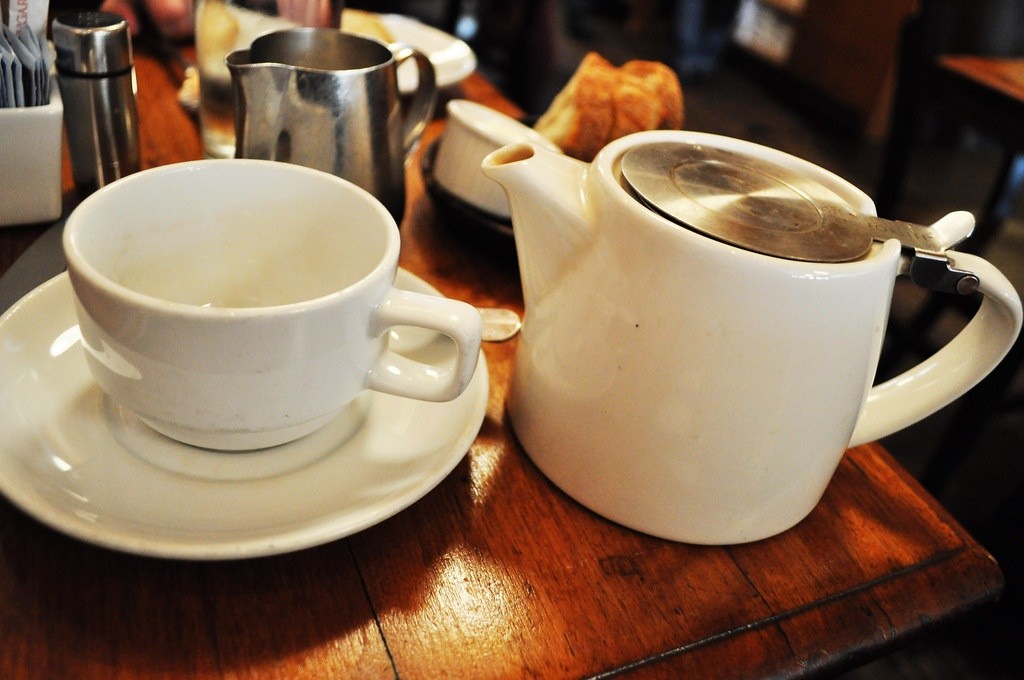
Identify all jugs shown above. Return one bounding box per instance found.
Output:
[483,129,1022,544]
[227,26,437,231]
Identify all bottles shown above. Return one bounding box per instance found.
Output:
[49,13,143,202]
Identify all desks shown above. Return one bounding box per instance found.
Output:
[0,63,1002,680]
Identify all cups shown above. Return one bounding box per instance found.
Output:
[57,157,482,450]
[0,70,65,223]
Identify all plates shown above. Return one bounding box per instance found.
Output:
[0,270,491,557]
[205,12,477,93]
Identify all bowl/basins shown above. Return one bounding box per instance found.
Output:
[431,99,558,217]
